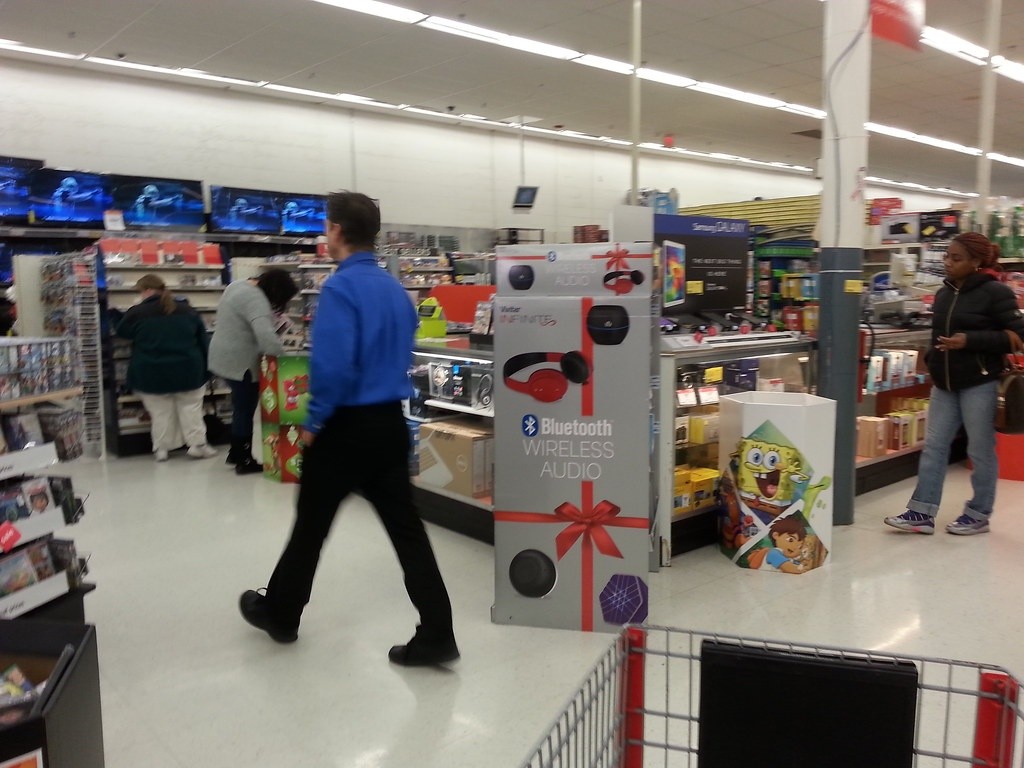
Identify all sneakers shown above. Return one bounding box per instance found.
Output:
[944,513,991,534]
[884,509,936,534]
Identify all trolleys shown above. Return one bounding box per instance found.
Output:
[517,619,1024,768]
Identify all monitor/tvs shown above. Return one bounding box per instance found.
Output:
[0,157,331,240]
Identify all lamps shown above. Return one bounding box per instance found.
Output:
[513,116,538,211]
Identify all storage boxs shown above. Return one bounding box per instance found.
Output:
[666,411,725,516]
[881,207,966,244]
[404,416,497,499]
[780,273,821,332]
[258,348,312,483]
[856,346,931,459]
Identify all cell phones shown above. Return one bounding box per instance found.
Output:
[663,241,685,308]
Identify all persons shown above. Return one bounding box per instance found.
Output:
[882,231,1023,537]
[238,190,461,667]
[108,273,216,458]
[208,267,300,475]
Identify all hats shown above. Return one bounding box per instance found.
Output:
[132,274,164,291]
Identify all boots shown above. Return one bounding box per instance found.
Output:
[225,440,237,463]
[235,433,263,474]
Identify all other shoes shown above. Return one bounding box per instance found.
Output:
[186,444,218,458]
[155,449,168,461]
[388,625,460,667]
[238,588,301,643]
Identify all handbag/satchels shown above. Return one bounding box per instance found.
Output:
[993,329,1024,434]
[203,414,234,446]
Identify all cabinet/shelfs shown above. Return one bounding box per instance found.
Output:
[755,241,821,319]
[93,237,238,458]
[0,619,107,768]
[297,261,457,353]
[657,340,820,559]
[853,324,969,496]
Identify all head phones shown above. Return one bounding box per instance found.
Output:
[602,270,644,293]
[503,351,591,402]
[475,372,493,405]
